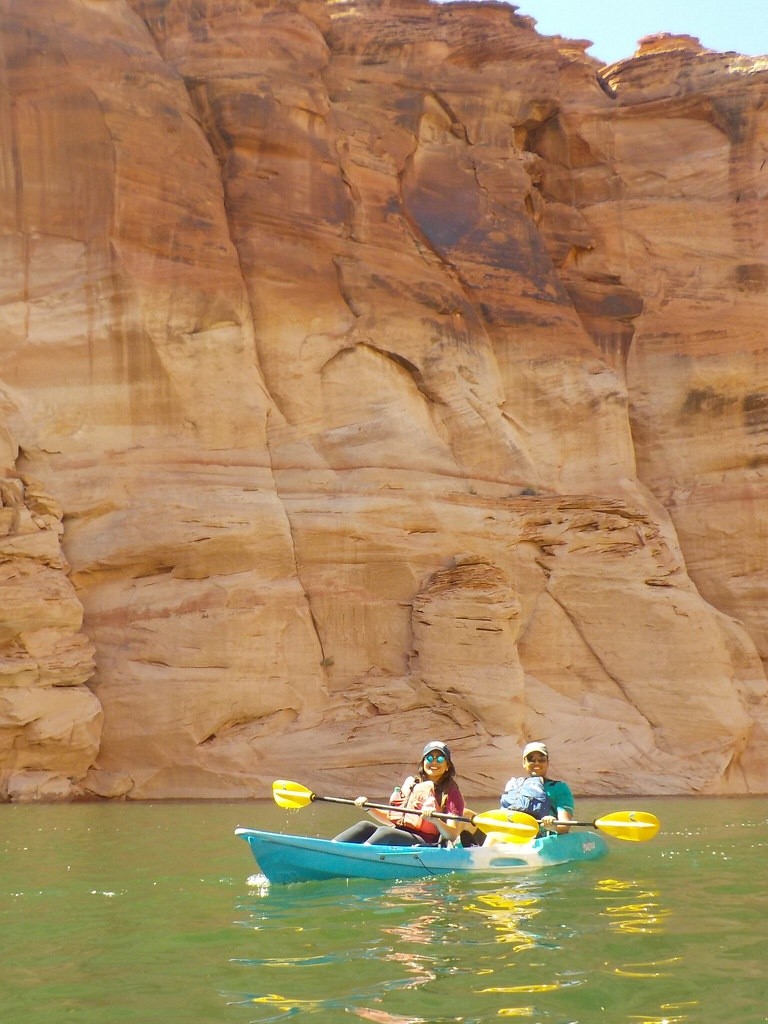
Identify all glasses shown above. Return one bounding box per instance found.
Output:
[424,755,446,764]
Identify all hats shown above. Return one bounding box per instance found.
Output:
[523,742,548,758]
[422,741,450,760]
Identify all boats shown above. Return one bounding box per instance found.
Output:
[235,828,607,886]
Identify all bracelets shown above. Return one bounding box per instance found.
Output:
[365,809,370,812]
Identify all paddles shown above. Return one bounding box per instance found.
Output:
[458,808,661,843]
[272,779,539,845]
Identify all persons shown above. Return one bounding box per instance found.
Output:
[459,741,575,849]
[331,741,466,845]
